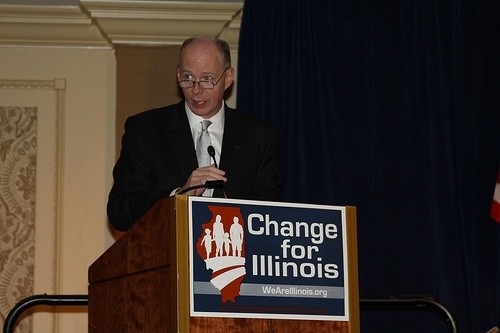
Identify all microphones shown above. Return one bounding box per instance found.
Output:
[208,146,226,199]
[178,180,224,194]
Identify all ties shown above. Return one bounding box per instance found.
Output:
[195,120,212,197]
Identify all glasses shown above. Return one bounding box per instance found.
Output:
[178,65,232,90]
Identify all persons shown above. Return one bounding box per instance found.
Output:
[107,36,281,232]
